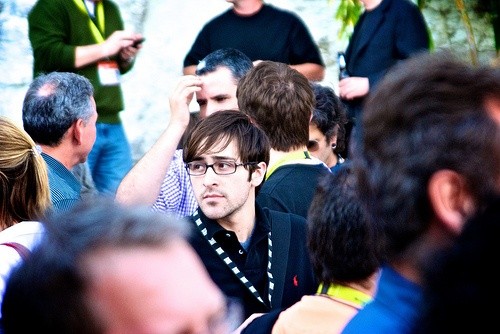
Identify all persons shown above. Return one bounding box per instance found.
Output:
[28,0,144,201]
[23,69,99,214]
[338,0,430,109]
[0,197,230,334]
[269,163,389,334]
[182,110,315,334]
[182,0,326,85]
[0,115,53,334]
[114,48,256,210]
[236,60,332,217]
[406,190,500,334]
[306,85,349,173]
[341,50,500,334]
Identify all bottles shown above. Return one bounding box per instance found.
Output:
[338,53,351,102]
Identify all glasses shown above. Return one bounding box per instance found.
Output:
[307,134,326,152]
[184,159,257,176]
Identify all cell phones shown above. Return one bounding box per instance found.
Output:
[131,37,146,48]
